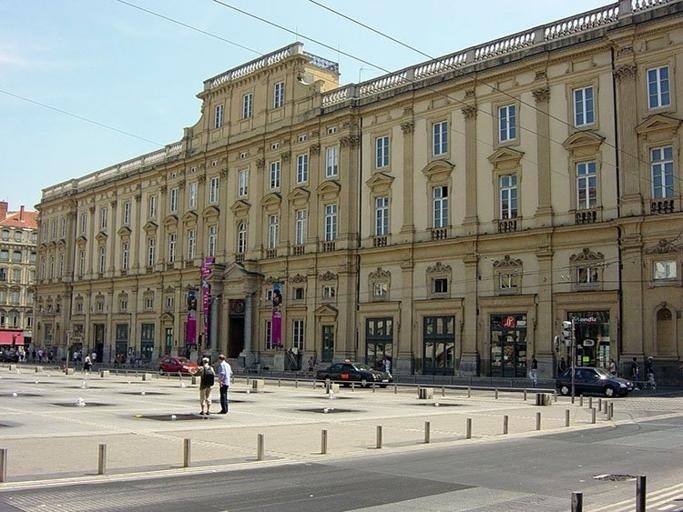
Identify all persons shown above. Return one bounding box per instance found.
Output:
[608,360,615,375]
[111,350,128,368]
[307,357,313,378]
[382,358,391,379]
[273,289,282,311]
[195,357,214,416]
[187,292,196,311]
[557,356,564,376]
[528,355,537,384]
[631,357,639,381]
[643,356,655,389]
[216,354,231,414]
[2,347,52,363]
[73,351,98,371]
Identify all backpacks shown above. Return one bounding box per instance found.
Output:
[201,366,214,386]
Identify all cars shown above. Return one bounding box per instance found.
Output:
[555,366,634,397]
[0,350,18,363]
[316,359,391,388]
[158,355,202,376]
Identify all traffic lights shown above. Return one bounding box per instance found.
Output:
[562,320,572,347]
